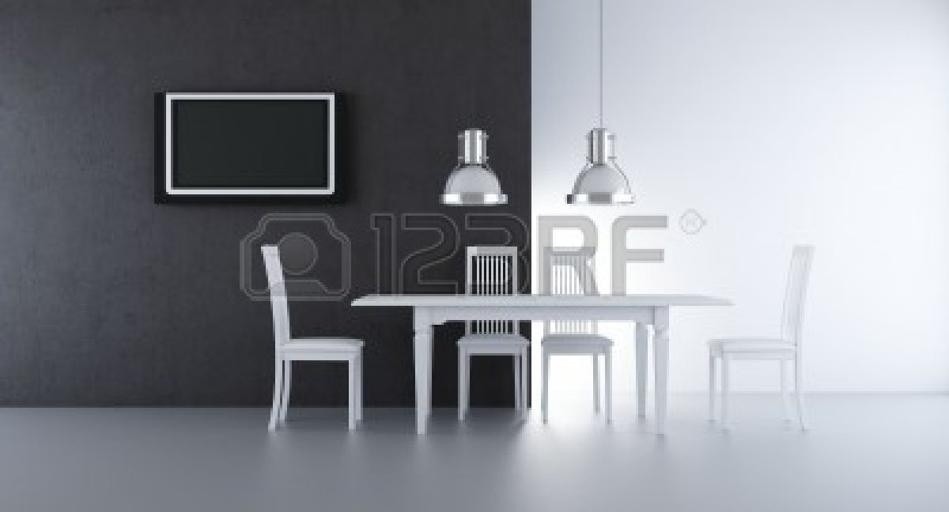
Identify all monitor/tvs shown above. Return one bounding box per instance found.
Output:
[154,88,346,205]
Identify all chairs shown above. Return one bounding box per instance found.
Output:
[253,242,367,431]
[708,242,813,429]
[460,244,614,428]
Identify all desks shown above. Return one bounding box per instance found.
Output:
[353,283,736,434]
